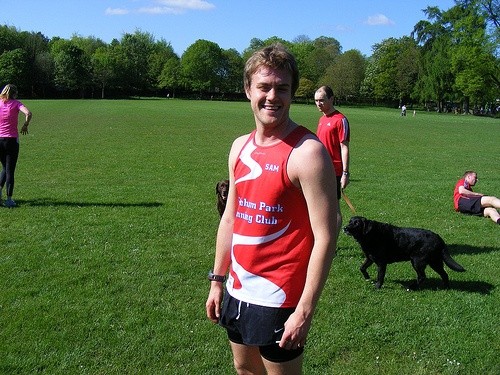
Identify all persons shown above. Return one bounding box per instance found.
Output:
[313,86,350,258]
[206,50,340,375]
[1,83,33,206]
[401,104,406,116]
[453,171,500,224]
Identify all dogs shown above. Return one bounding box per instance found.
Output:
[214,179,229,217]
[343,216,467,290]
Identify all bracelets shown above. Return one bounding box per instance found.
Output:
[343,170,348,175]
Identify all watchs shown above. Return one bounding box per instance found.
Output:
[207,270,226,282]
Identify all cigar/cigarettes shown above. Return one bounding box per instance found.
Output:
[276,341,281,343]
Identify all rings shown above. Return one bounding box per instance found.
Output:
[289,342,293,344]
[295,343,300,347]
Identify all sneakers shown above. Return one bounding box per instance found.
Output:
[6,199,16,205]
